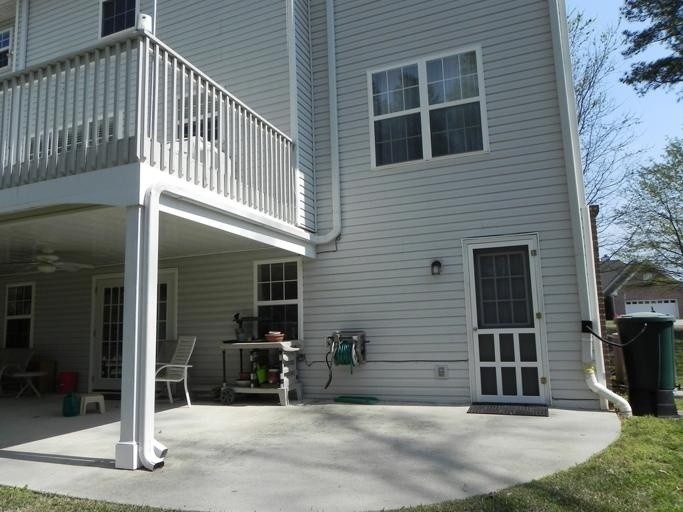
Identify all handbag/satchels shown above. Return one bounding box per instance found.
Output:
[63,392,79,416]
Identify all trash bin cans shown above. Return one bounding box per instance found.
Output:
[614,313,675,391]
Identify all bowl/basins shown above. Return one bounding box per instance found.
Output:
[234,380,250,387]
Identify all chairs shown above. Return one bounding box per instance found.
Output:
[155,335,197,407]
[0,348,36,398]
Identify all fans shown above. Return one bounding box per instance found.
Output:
[0,253,96,276]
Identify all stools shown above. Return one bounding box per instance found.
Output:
[78,393,106,415]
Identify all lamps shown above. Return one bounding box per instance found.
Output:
[431,261,441,275]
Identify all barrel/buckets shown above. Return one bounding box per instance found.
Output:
[256,367,267,383]
[56,372,77,393]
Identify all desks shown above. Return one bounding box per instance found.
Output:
[11,372,48,399]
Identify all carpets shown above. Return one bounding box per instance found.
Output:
[466,401,548,417]
